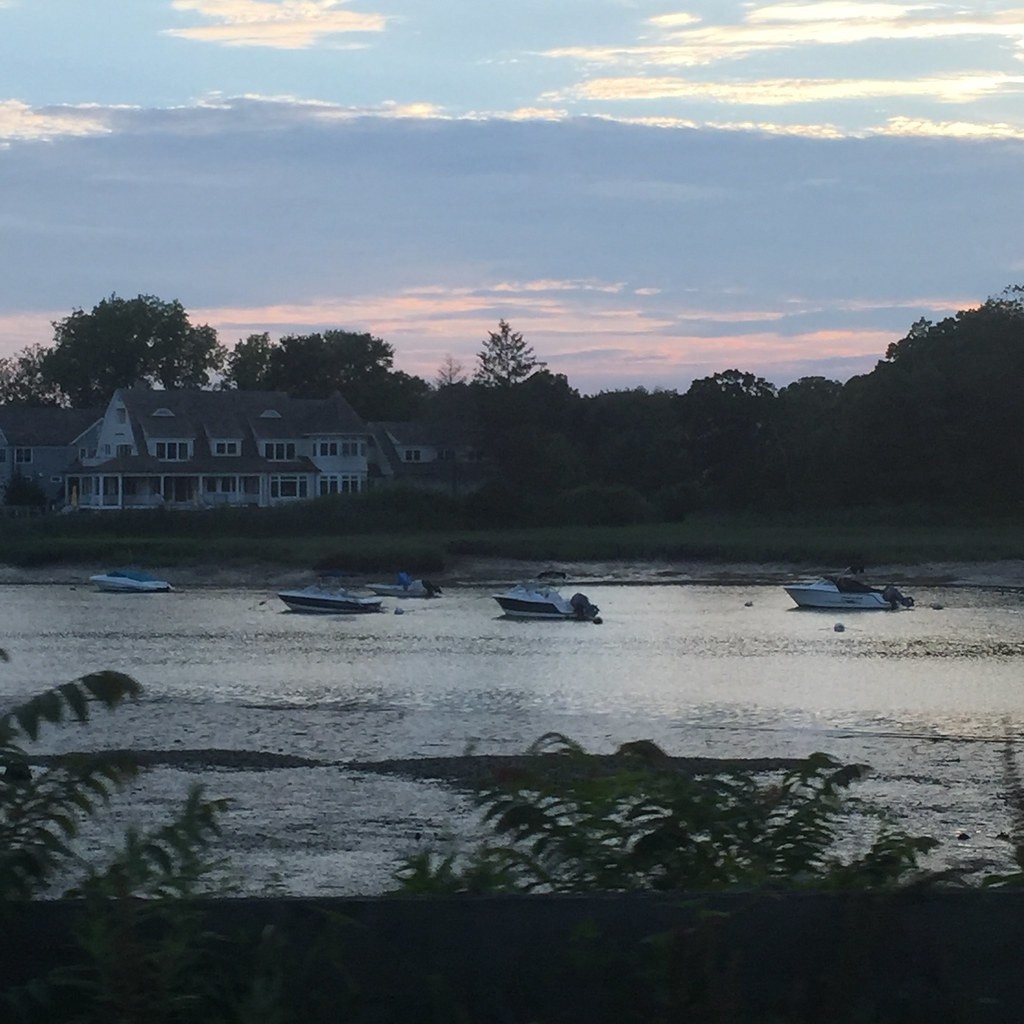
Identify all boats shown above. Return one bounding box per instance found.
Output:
[90,571,175,593]
[782,574,900,609]
[491,581,600,620]
[278,577,385,613]
[364,579,443,597]
